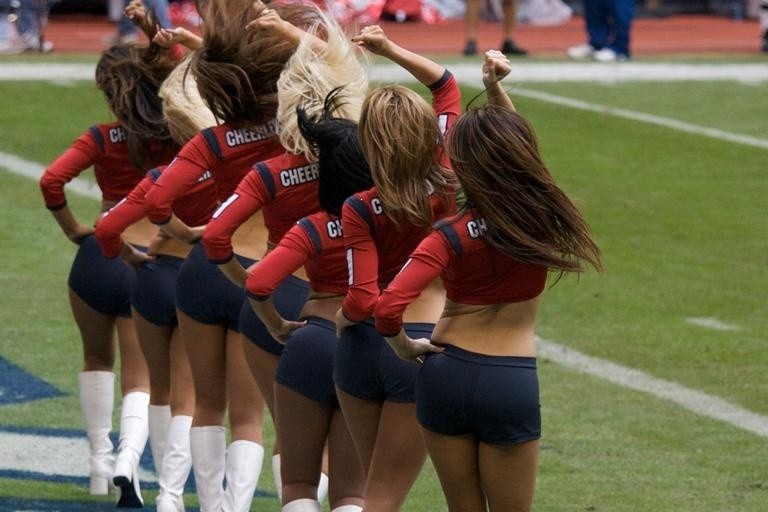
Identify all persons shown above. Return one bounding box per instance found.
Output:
[94,25,226,509]
[35,0,183,510]
[198,7,370,511]
[329,25,462,511]
[143,0,329,510]
[462,1,528,56]
[373,47,605,512]
[1,1,55,55]
[240,84,371,511]
[120,1,171,36]
[747,1,766,51]
[567,1,639,61]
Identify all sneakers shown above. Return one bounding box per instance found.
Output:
[462,37,629,63]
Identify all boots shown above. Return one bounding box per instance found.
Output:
[71,372,114,497]
[268,453,368,512]
[147,388,193,512]
[187,425,228,512]
[112,385,151,510]
[222,438,264,512]
